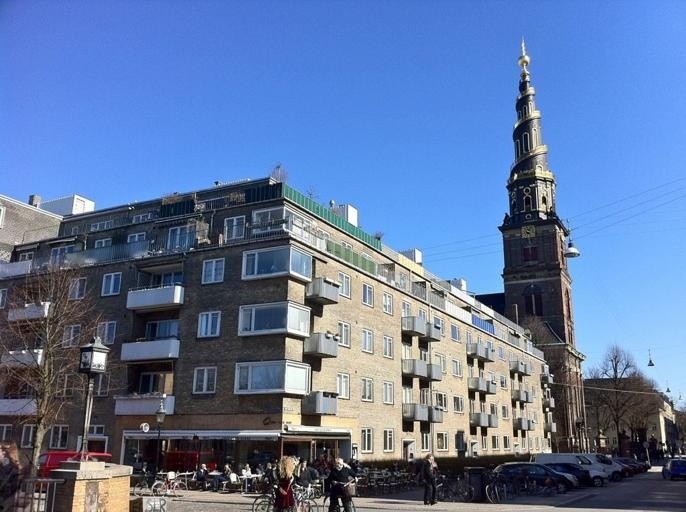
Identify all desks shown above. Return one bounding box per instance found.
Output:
[155,471,261,495]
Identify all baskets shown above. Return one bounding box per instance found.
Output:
[341,484,355,497]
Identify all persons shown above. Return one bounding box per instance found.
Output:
[418,453,438,506]
[647,433,677,463]
[196,450,359,512]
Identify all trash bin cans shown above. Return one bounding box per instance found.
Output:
[464,467,484,500]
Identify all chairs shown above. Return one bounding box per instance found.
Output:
[353,466,415,498]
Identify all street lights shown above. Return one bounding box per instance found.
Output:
[68,335,111,461]
[152,398,169,486]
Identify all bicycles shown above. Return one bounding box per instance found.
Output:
[251,479,361,512]
[137,471,187,498]
[436,473,559,503]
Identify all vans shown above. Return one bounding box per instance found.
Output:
[19,451,113,493]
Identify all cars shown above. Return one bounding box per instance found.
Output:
[493,453,651,492]
[661,458,685,482]
